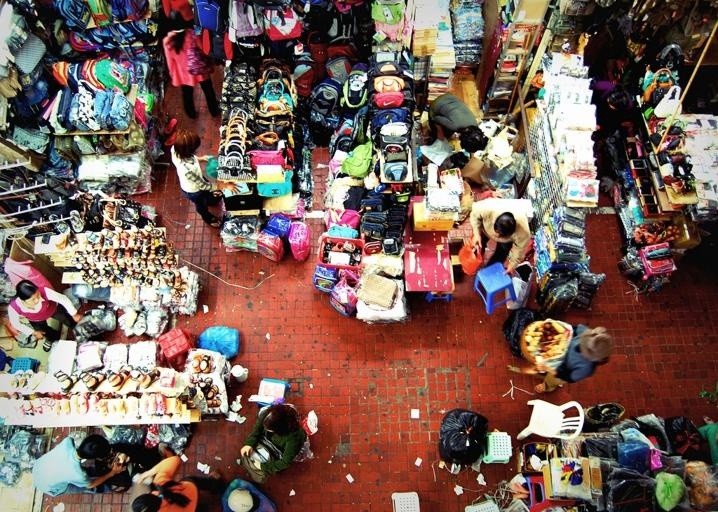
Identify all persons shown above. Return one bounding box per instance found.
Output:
[170,129,223,229]
[161,9,221,118]
[521,323,612,394]
[591,87,633,151]
[130,455,226,512]
[7,279,83,352]
[220,479,278,512]
[427,92,488,153]
[30,430,124,497]
[469,197,534,295]
[237,403,305,485]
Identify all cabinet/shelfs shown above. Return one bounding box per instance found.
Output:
[612,2,718,291]
[55,86,151,160]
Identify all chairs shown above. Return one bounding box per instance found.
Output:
[516,399,585,441]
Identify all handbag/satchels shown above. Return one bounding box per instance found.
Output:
[216,30,359,211]
[312,265,398,318]
[367,41,417,181]
[1,0,162,223]
[543,0,718,55]
[636,43,685,133]
[256,212,311,263]
[186,30,214,76]
[97,198,157,230]
[360,183,410,255]
[458,242,483,276]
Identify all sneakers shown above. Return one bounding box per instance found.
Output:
[49,210,182,290]
[42,339,52,352]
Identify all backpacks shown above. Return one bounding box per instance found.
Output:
[242,430,284,485]
[192,0,410,49]
[502,308,544,357]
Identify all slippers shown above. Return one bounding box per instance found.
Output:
[208,218,222,229]
[164,118,178,134]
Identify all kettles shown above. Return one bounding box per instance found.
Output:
[229,365,248,383]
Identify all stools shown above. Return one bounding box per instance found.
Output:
[7,357,41,375]
[255,378,292,408]
[390,491,420,512]
[482,431,513,465]
[157,328,195,371]
[523,476,577,512]
[464,499,501,512]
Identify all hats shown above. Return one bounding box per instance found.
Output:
[227,488,254,512]
[578,325,614,364]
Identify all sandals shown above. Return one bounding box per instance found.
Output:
[10,354,222,419]
